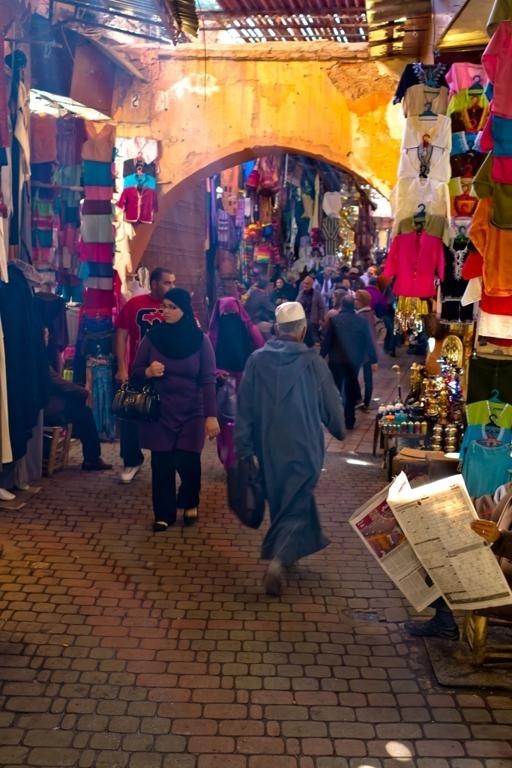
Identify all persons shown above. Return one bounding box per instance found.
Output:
[207,296,265,472]
[240,274,302,341]
[355,289,376,409]
[0,262,49,509]
[132,288,221,532]
[81,320,121,441]
[319,288,348,342]
[34,282,69,375]
[42,343,113,472]
[294,275,328,347]
[319,295,379,431]
[404,481,512,640]
[115,267,201,484]
[309,264,396,358]
[233,300,347,595]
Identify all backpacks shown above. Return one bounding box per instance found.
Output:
[374,316,388,345]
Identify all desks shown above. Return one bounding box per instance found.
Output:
[372,415,426,469]
[390,447,460,479]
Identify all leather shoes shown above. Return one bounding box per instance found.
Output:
[81,458,113,470]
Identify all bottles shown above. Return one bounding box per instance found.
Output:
[378,400,429,437]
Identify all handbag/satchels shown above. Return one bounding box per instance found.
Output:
[112,384,162,422]
[227,464,265,530]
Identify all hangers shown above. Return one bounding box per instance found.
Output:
[62,109,76,120]
[405,61,485,245]
[476,389,505,447]
[85,312,119,368]
[136,125,147,191]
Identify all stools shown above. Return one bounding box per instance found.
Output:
[460,610,511,667]
[42,423,73,477]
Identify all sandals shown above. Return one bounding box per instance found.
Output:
[153,506,173,532]
[183,505,200,527]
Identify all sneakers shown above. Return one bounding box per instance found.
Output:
[1,486,42,509]
[119,465,140,483]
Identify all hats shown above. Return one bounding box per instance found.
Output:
[275,301,306,324]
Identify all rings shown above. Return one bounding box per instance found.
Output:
[482,529,485,534]
